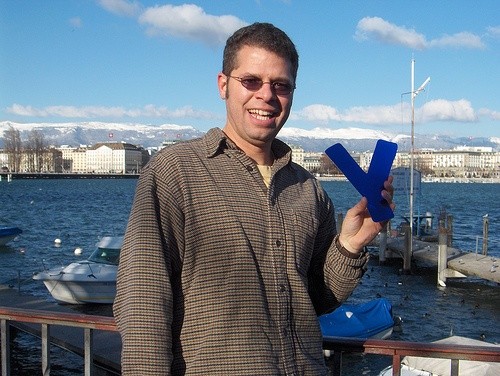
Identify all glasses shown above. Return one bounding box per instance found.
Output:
[228,75,294,95]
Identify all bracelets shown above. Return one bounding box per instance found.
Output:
[336,241,367,258]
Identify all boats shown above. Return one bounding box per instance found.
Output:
[0,225,22,247]
[32,235,125,304]
[316,297,403,341]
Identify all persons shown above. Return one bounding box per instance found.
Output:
[113,22,396,376]
